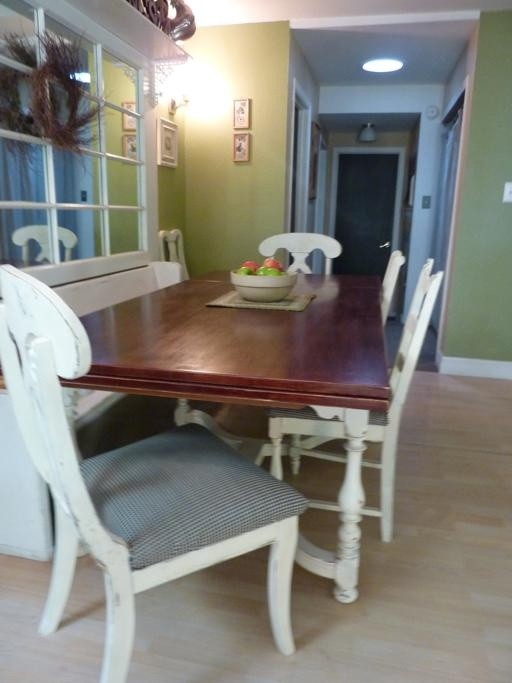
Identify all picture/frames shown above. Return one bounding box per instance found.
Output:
[233,99,250,129]
[122,102,136,131]
[122,134,136,160]
[309,120,320,200]
[232,132,249,162]
[156,117,179,168]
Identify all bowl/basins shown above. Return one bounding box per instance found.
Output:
[229,267,299,300]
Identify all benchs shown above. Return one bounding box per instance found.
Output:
[0,250,181,562]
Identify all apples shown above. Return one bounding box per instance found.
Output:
[235,258,288,276]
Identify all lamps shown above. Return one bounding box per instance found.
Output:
[357,122,377,142]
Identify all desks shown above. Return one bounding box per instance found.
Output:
[0,272,391,603]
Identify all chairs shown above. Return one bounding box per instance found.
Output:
[267,270,444,543]
[259,232,343,274]
[11,225,78,267]
[1,263,308,683]
[380,248,406,322]
[159,228,190,280]
